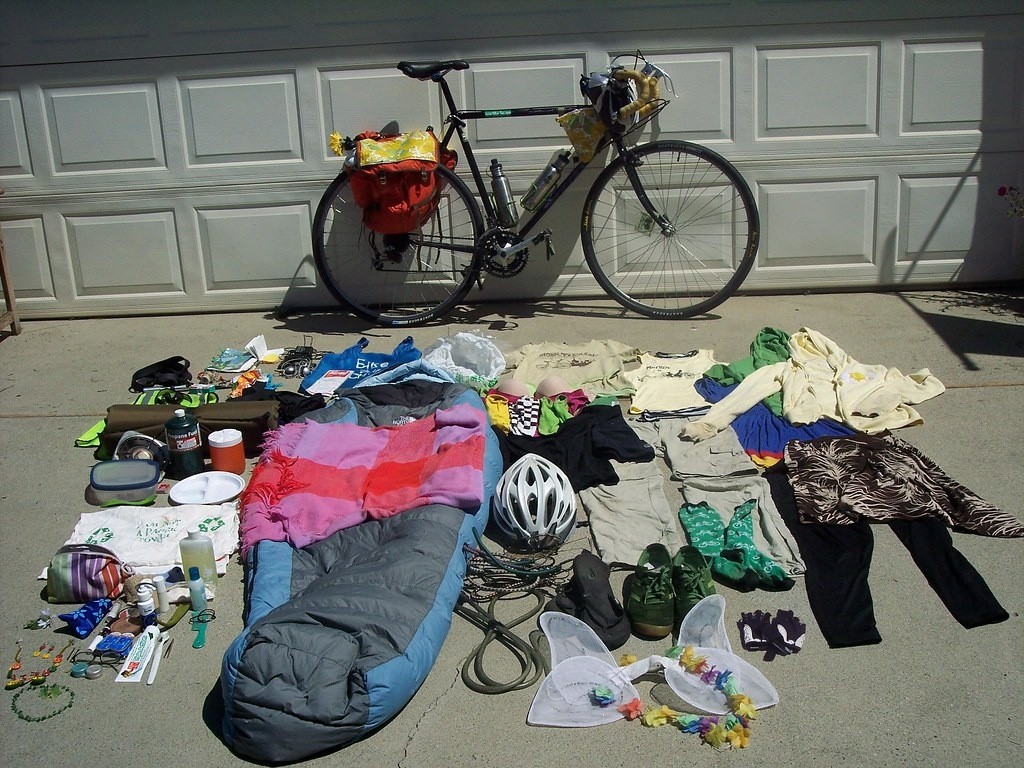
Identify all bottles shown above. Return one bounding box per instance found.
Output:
[179,527,218,585]
[485,159,520,227]
[522,150,571,209]
[164,409,204,481]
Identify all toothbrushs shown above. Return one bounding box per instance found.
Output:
[147,627,170,686]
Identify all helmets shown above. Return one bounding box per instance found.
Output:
[491,452,577,546]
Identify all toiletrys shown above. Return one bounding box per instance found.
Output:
[114,625,160,683]
[151,576,170,614]
[184,566,211,611]
[179,526,218,591]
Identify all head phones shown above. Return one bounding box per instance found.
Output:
[274,357,311,379]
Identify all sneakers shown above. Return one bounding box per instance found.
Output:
[672,544,722,640]
[627,543,674,637]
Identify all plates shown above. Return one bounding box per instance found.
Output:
[170,471,245,505]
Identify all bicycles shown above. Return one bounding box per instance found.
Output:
[310,46,761,329]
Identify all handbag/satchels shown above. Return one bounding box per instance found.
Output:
[348,127,461,237]
